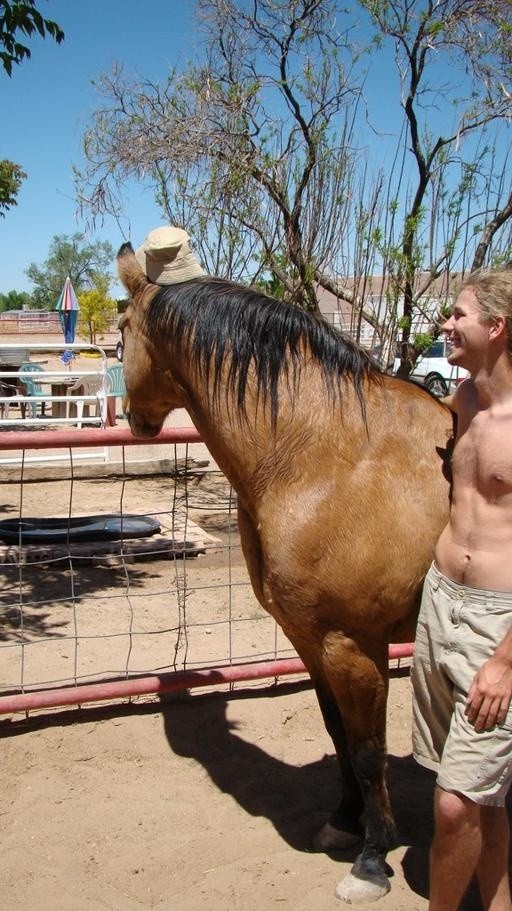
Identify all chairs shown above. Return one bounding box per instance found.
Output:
[66,372,111,429]
[17,364,52,418]
[0,380,28,419]
[106,363,129,420]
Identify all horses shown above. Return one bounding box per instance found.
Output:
[113,235,461,909]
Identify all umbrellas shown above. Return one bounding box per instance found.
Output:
[55,276,81,365]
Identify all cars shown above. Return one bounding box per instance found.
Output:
[360,336,472,403]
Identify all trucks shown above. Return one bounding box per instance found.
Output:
[114,296,132,362]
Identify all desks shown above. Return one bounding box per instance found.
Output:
[32,377,90,417]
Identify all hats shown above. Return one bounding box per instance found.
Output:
[134,226,205,287]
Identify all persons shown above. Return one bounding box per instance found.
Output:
[410,265,512,910]
